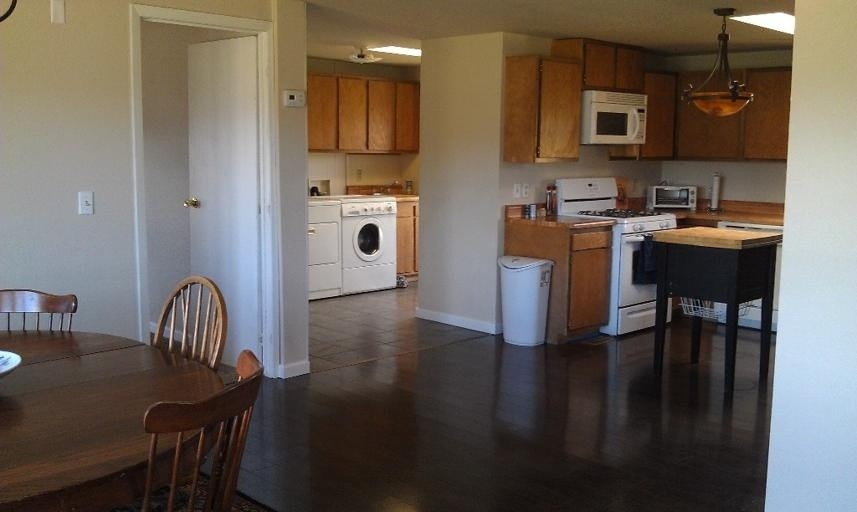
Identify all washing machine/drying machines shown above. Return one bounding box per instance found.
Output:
[341,199,397,295]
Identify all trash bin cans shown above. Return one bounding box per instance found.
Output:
[497,255,555,346]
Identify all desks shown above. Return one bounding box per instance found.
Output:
[649,225,784,407]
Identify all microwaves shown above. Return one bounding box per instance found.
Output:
[582,90,647,145]
[648,186,698,210]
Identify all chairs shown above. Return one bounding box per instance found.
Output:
[151,274,228,374]
[0,288,78,334]
[138,349,265,512]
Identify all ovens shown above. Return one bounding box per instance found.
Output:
[600,221,677,337]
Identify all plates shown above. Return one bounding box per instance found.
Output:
[0,351,21,374]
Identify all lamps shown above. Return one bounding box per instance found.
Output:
[681,7,757,120]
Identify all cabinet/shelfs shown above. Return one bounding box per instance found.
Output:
[552,33,678,163]
[344,182,418,281]
[642,63,791,163]
[503,53,584,163]
[306,71,421,159]
[503,202,617,350]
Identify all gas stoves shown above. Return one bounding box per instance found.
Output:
[555,177,675,224]
